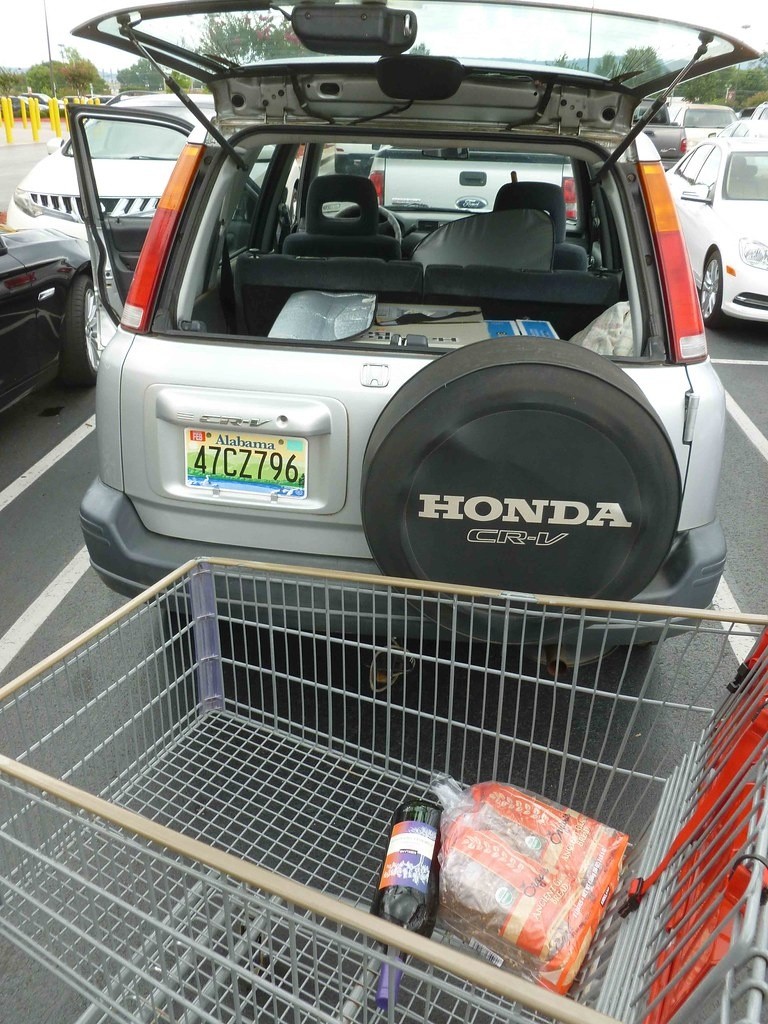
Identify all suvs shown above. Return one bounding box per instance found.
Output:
[63,0,760,672]
[5,90,227,292]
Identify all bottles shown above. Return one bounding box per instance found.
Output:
[369,800,444,1009]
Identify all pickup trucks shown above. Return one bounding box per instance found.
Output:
[629,98,687,171]
[366,143,583,236]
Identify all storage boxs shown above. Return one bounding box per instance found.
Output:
[517,318,560,340]
[485,320,521,340]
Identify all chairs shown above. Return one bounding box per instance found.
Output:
[494,181,589,272]
[729,157,763,197]
[282,174,402,261]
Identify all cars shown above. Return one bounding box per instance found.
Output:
[663,138,768,326]
[6,94,65,118]
[708,120,768,140]
[0,219,102,417]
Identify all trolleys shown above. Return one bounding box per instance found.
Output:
[0,556,768,1024]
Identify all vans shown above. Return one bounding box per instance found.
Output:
[668,103,739,160]
